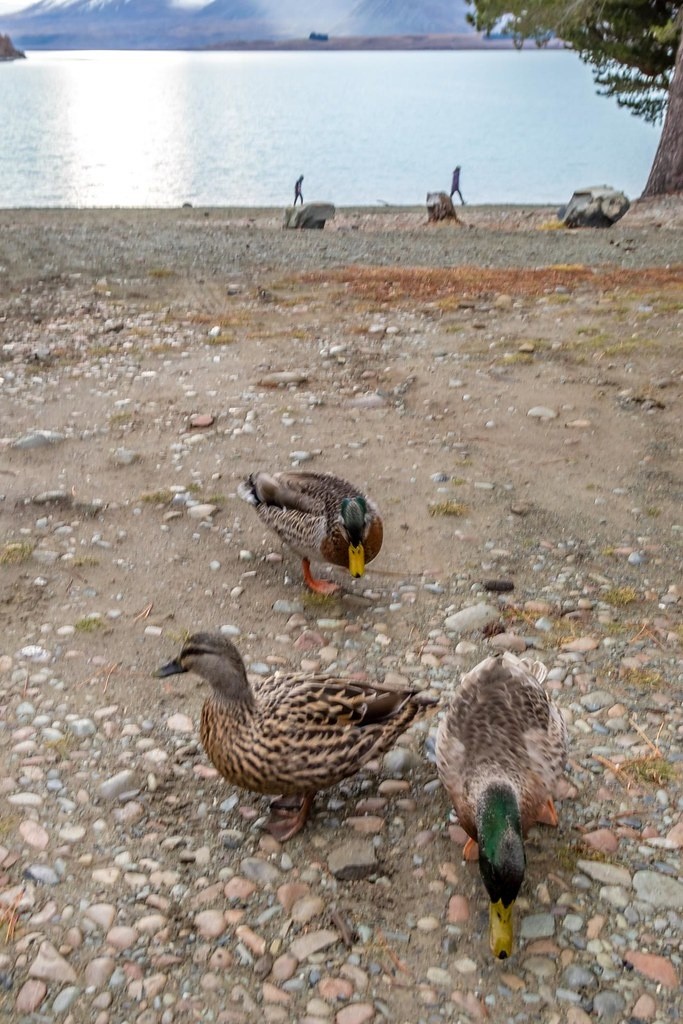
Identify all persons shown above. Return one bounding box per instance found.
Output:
[294,176,304,207]
[451,166,468,206]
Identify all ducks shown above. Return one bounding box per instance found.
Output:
[238,469,383,594]
[152,631,452,843]
[435,651,578,958]
